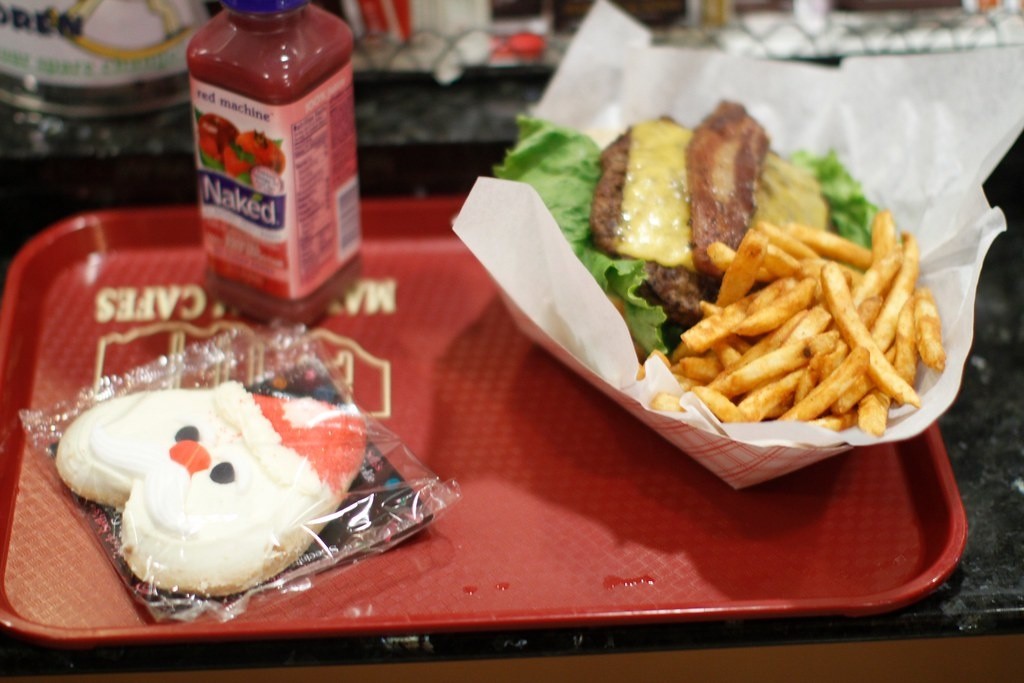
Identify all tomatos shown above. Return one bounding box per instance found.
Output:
[224,131,285,180]
[199,113,237,160]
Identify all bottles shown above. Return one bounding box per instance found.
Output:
[185,0,364,327]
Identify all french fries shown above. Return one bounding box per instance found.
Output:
[637,216,945,438]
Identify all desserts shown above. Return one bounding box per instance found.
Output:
[54,386,366,596]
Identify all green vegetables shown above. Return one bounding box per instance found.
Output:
[795,152,876,246]
[491,119,668,363]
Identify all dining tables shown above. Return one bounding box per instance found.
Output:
[0,56,1024,673]
